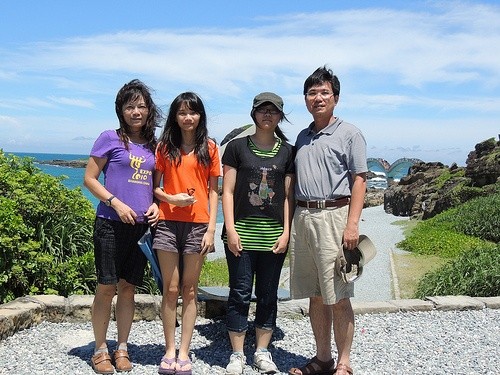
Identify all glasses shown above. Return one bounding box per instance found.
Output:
[256,108,279,114]
[306,90,334,99]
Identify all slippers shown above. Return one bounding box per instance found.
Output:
[158,357,176,374]
[175,358,192,375]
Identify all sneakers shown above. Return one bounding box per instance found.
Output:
[225,352,246,375]
[252,349,279,373]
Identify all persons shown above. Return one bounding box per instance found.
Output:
[289,66,368,375]
[222,92,295,375]
[84,80,160,374]
[151,92,220,375]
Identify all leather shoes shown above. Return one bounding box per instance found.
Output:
[114,350,132,371]
[91,352,114,374]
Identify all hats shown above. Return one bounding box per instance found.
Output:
[253,92,284,111]
[336,235,377,283]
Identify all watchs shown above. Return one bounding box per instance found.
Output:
[105,196,115,206]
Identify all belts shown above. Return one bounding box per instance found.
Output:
[298,197,350,209]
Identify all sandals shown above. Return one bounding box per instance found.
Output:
[289,356,336,375]
[332,363,353,375]
